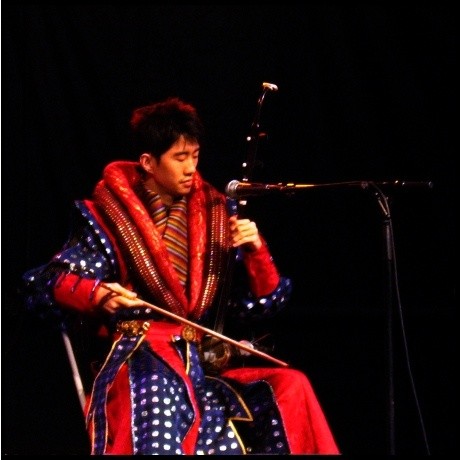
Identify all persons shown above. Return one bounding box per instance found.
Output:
[20,97,343,455]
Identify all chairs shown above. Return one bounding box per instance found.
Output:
[46,252,108,460]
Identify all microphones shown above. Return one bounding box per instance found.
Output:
[227,179,295,199]
